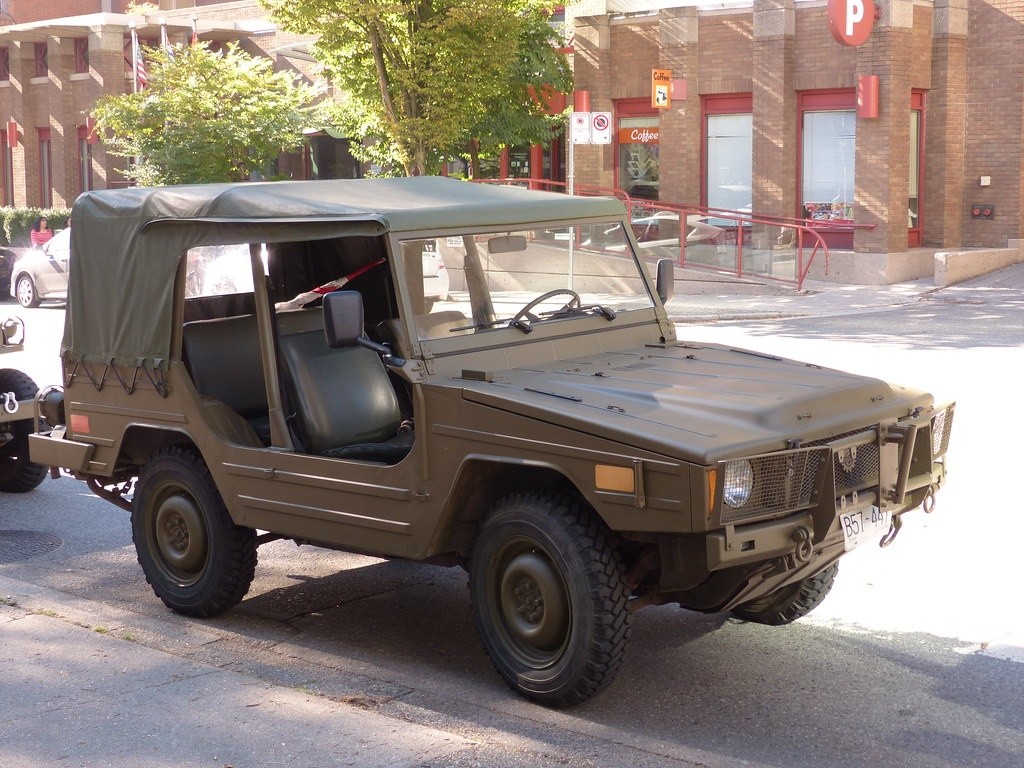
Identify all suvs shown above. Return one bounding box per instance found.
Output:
[0,311,52,493]
[25,173,960,713]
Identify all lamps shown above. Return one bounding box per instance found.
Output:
[6,121,19,148]
[87,117,100,145]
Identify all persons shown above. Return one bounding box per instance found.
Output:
[30,216,53,248]
[61,216,71,230]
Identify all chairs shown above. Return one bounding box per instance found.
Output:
[183,302,471,457]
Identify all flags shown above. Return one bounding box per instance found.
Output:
[134,33,149,94]
[276,256,385,311]
[192,21,201,48]
[162,28,175,68]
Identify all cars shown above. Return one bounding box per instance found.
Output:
[9,225,209,310]
[0,248,18,304]
[198,235,450,314]
[624,181,660,200]
